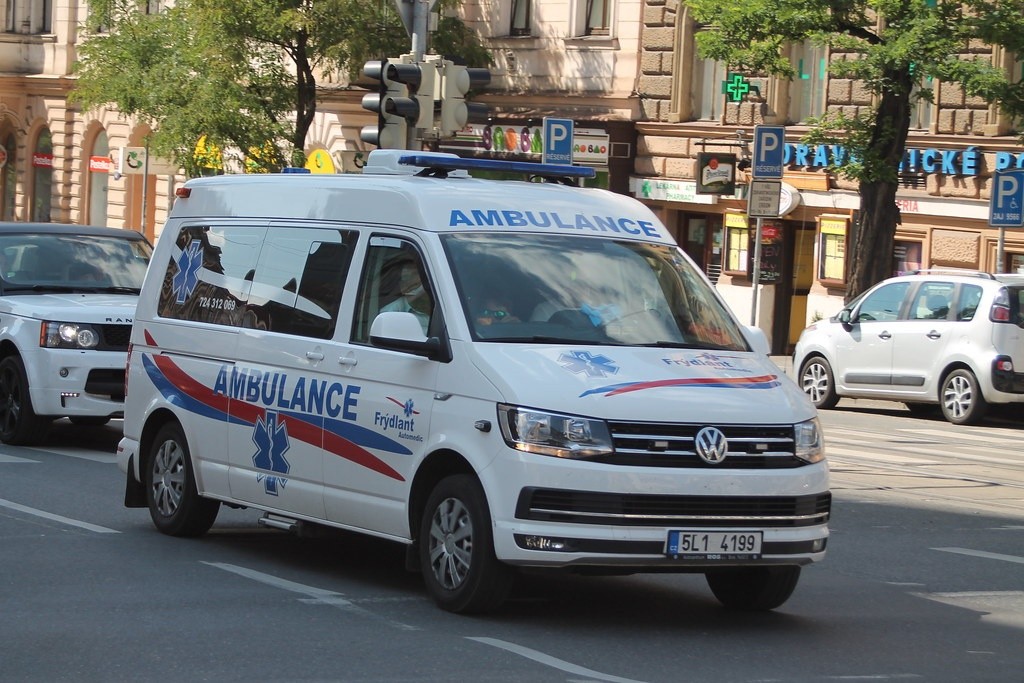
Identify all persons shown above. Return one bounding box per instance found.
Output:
[529,298,568,322]
[76,261,109,281]
[379,262,432,337]
[481,294,522,323]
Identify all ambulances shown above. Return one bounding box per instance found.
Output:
[117,149,831,612]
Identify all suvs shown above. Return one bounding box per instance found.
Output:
[0,222,155,446]
[792,268,1024,425]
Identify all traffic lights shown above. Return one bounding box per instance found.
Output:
[440,60,491,131]
[360,58,401,150]
[385,62,435,127]
